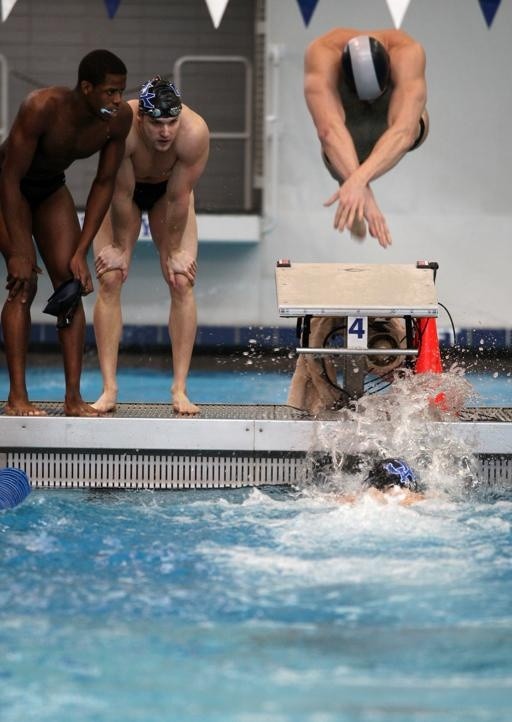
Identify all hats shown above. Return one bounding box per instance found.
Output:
[369,458,416,489]
[342,35,389,101]
[139,78,182,118]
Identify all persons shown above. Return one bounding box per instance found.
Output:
[0,49,134,417]
[302,27,429,249]
[86,73,211,415]
[310,450,425,508]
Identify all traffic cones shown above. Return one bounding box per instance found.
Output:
[402,314,463,415]
